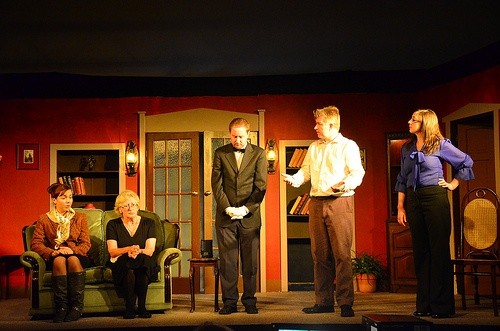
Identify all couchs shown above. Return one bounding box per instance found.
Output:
[21,208,182,316]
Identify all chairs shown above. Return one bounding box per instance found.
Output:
[451,188,500,317]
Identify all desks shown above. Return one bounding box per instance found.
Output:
[0,255,29,300]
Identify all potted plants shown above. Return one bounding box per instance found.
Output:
[350,251,385,293]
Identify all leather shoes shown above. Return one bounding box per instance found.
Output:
[219,305,237,314]
[123,309,135,319]
[303,303,335,313]
[138,308,151,318]
[414,311,431,316]
[245,305,258,313]
[431,312,455,318]
[341,304,354,317]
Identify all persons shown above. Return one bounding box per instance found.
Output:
[31,183,91,322]
[281,106,365,317]
[211,117,267,314]
[106,190,156,319]
[395,108,474,318]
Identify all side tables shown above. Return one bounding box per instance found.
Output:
[188,256,220,312]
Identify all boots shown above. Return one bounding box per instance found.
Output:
[63,271,86,321]
[51,276,68,323]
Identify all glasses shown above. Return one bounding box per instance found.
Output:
[120,202,137,209]
[410,118,423,124]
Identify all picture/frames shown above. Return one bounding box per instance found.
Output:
[16,143,40,170]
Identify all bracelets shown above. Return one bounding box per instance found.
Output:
[141,250,144,254]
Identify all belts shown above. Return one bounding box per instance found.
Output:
[313,195,339,200]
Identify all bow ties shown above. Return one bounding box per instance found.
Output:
[232,146,245,153]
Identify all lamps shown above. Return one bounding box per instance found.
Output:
[126,141,139,175]
[266,138,280,173]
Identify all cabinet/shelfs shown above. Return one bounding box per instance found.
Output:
[279,140,312,239]
[56,149,119,212]
[388,140,406,215]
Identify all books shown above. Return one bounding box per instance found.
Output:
[59,176,86,195]
[289,193,310,215]
[288,148,308,168]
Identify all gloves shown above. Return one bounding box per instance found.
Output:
[225,205,248,219]
